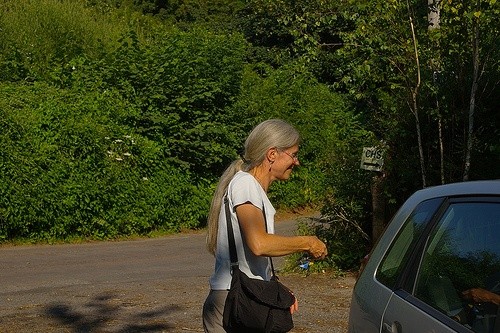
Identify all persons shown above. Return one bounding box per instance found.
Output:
[202,119,328,333]
[454,280,500,333]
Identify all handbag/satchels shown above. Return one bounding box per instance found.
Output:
[223,269,295,333]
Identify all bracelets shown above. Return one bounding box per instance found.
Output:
[454,316,460,323]
[271,276,280,281]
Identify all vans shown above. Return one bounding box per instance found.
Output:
[346,180,500,333]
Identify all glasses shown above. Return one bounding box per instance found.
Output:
[276,146,298,162]
